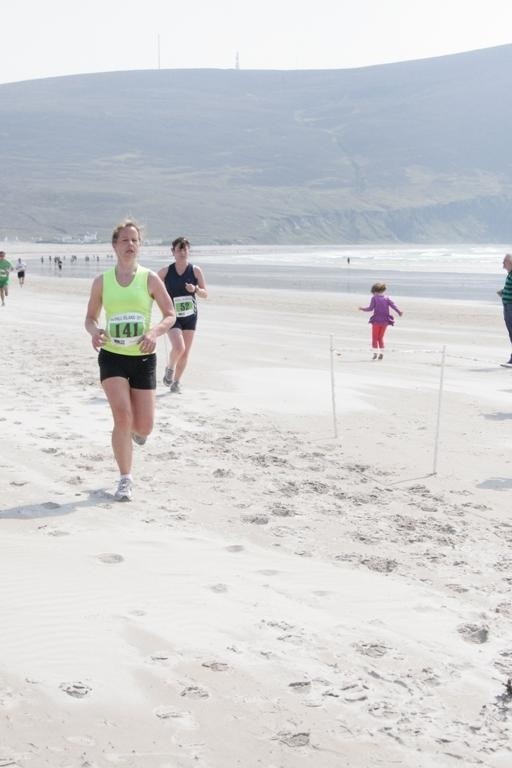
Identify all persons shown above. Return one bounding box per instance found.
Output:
[496,253,512,367]
[0,250,27,306]
[358,283,404,360]
[158,237,209,395]
[85,220,177,502]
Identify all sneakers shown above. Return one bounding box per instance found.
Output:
[115,477,134,502]
[500,361,512,367]
[132,433,147,445]
[163,366,182,395]
